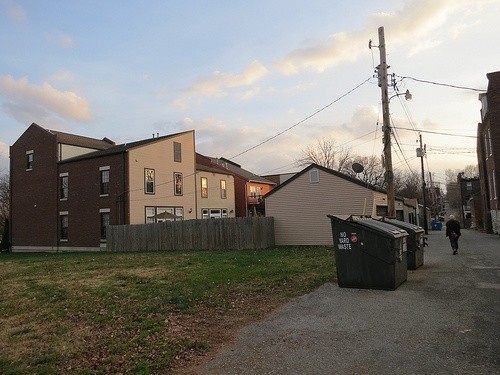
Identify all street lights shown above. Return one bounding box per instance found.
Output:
[383,89,412,218]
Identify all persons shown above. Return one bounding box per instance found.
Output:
[446,214,462,255]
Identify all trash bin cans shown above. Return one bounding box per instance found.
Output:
[327,213,409,291]
[371,215,426,270]
[431,222,442,231]
